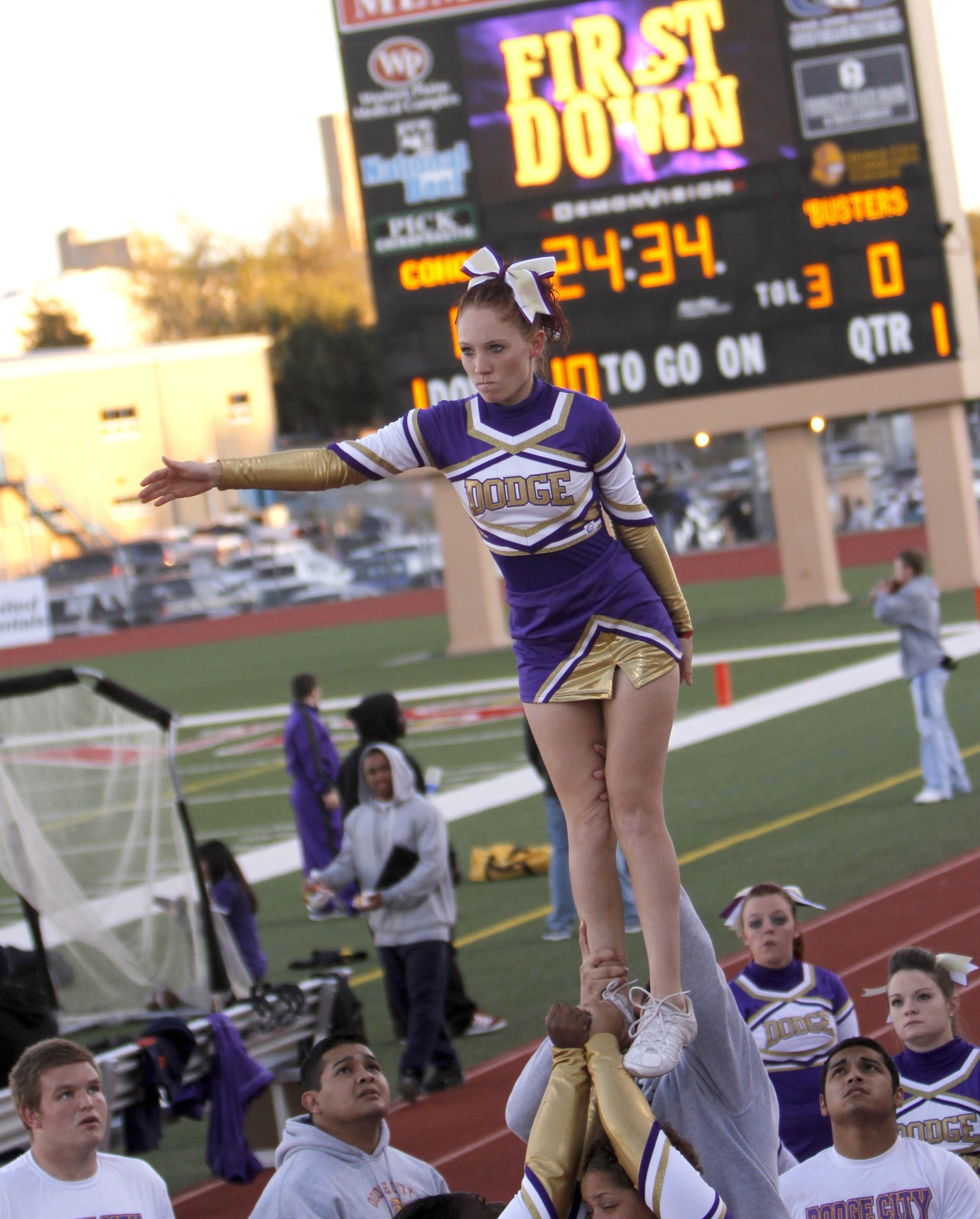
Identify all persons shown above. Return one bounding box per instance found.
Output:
[141,248,698,1076]
[386,743,800,1219]
[887,947,980,1179]
[719,883,855,1167]
[244,1033,451,1219]
[155,839,268,1008]
[0,1040,176,1219]
[869,543,972,804]
[282,671,364,922]
[301,741,464,1100]
[775,1037,980,1219]
[338,691,506,1046]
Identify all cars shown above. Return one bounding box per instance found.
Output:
[42,519,442,635]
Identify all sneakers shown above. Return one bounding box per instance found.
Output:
[622,987,698,1078]
[601,979,635,1027]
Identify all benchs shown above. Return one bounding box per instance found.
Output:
[0,965,354,1167]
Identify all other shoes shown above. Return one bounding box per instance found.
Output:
[310,908,343,921]
[464,1012,506,1034]
[397,1076,418,1102]
[914,790,950,804]
[424,1072,462,1092]
